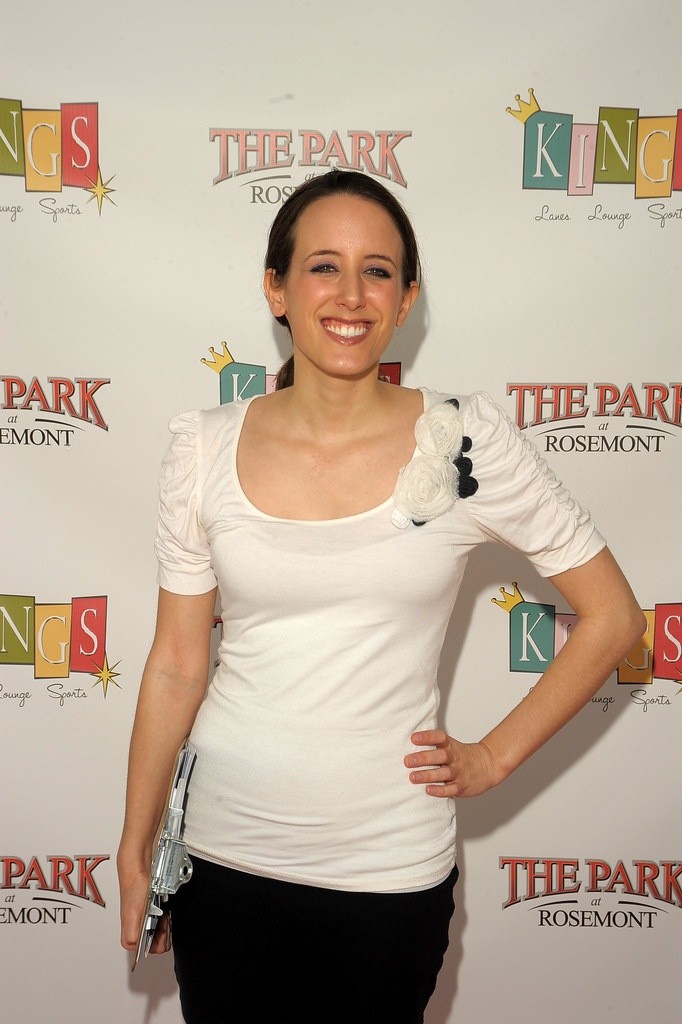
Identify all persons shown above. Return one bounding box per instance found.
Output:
[115,169,646,1024]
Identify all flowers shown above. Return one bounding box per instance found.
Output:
[394,402,466,524]
